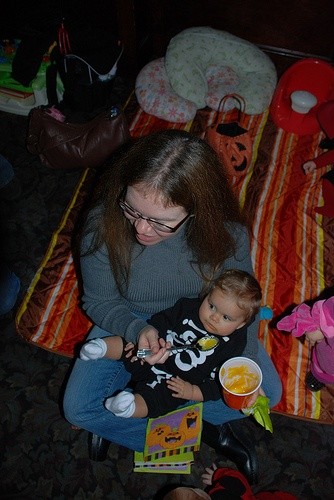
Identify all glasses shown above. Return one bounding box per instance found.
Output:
[121,185,194,233]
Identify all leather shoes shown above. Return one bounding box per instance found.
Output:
[214,421,259,488]
[88,432,112,462]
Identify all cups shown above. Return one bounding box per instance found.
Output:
[219,356,262,410]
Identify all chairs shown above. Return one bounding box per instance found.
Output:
[12,44,334,425]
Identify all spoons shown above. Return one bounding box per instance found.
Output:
[136,335,220,358]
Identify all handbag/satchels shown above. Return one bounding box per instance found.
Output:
[204,91,254,202]
[18,101,134,175]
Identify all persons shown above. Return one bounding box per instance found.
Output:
[303,102,334,218]
[61,128,282,481]
[163,462,298,500]
[304,296,334,392]
[79,269,263,419]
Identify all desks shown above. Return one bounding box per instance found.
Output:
[0,87,36,137]
